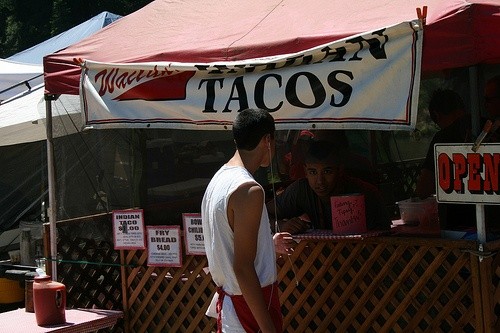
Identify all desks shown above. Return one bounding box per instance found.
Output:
[0,304,125,333]
[146,175,211,199]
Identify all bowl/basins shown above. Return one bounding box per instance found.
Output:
[395,197,440,228]
[8,251,20,263]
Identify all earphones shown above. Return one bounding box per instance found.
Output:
[268,142,270,147]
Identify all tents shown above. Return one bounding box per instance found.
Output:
[0,0,500,280]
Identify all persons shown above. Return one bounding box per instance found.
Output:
[201,108,293,333]
[266,136,393,236]
[417,90,477,232]
[483,74,500,142]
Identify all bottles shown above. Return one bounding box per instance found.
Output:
[24,272,39,312]
[33,276,66,327]
